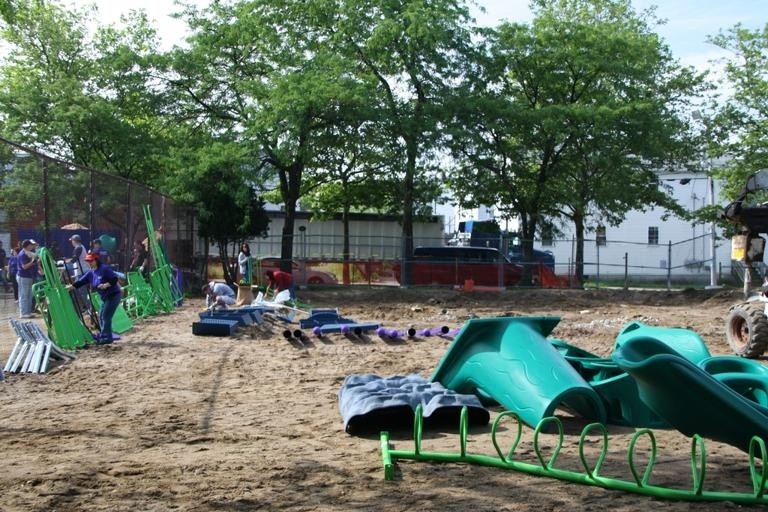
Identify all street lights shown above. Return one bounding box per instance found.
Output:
[691,108,724,289]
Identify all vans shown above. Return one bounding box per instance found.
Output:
[394,246,524,286]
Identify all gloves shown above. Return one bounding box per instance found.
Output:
[140,266,144,272]
[57,260,65,266]
[32,255,38,263]
[6,273,11,281]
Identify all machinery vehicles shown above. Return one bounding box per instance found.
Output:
[716,167,768,359]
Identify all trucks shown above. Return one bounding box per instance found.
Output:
[456,219,555,284]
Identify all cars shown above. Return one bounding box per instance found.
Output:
[446,234,459,246]
[223,257,337,286]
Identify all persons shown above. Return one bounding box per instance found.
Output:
[201,283,237,314]
[238,243,256,281]
[263,269,295,304]
[0,235,149,344]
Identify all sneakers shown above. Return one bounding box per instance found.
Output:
[21,313,34,318]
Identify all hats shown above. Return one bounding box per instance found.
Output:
[69,235,81,241]
[22,238,36,247]
[84,254,98,261]
